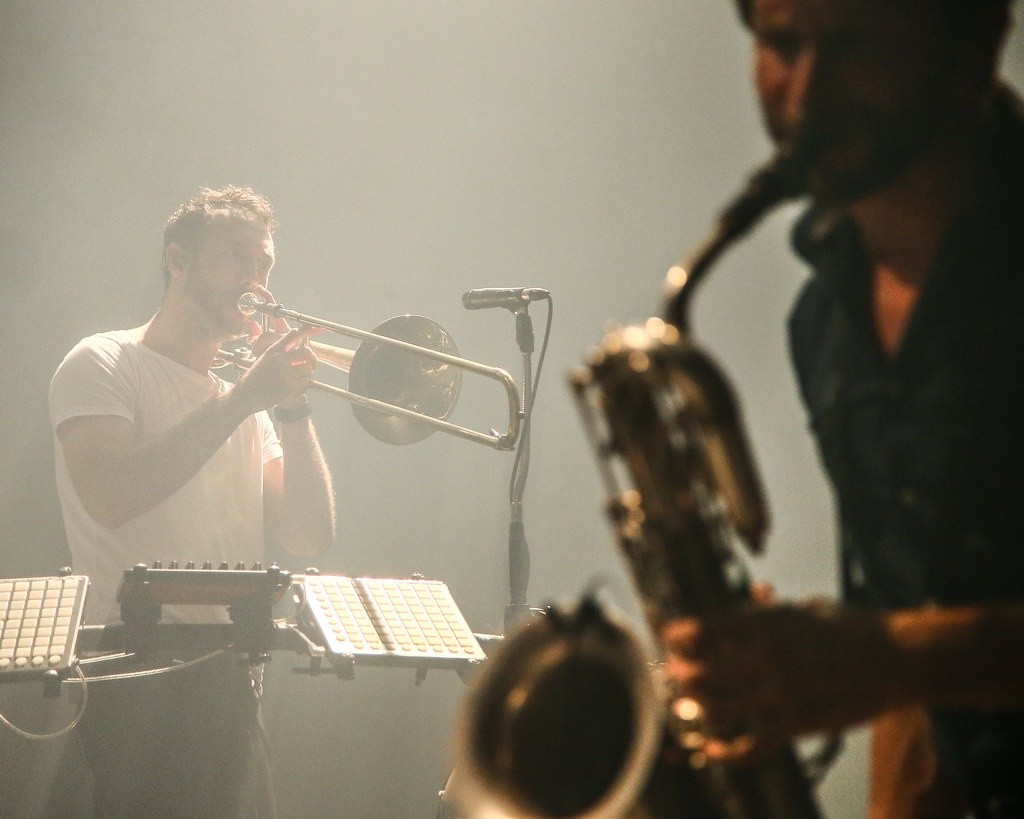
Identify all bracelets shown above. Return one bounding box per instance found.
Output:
[271,401,310,422]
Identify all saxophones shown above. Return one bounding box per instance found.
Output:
[447,144,828,819]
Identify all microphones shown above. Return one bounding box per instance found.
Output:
[462,287,550,310]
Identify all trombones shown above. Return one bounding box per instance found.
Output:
[215,291,524,452]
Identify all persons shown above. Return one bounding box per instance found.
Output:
[47,184,336,819]
[658,0,1023,819]
